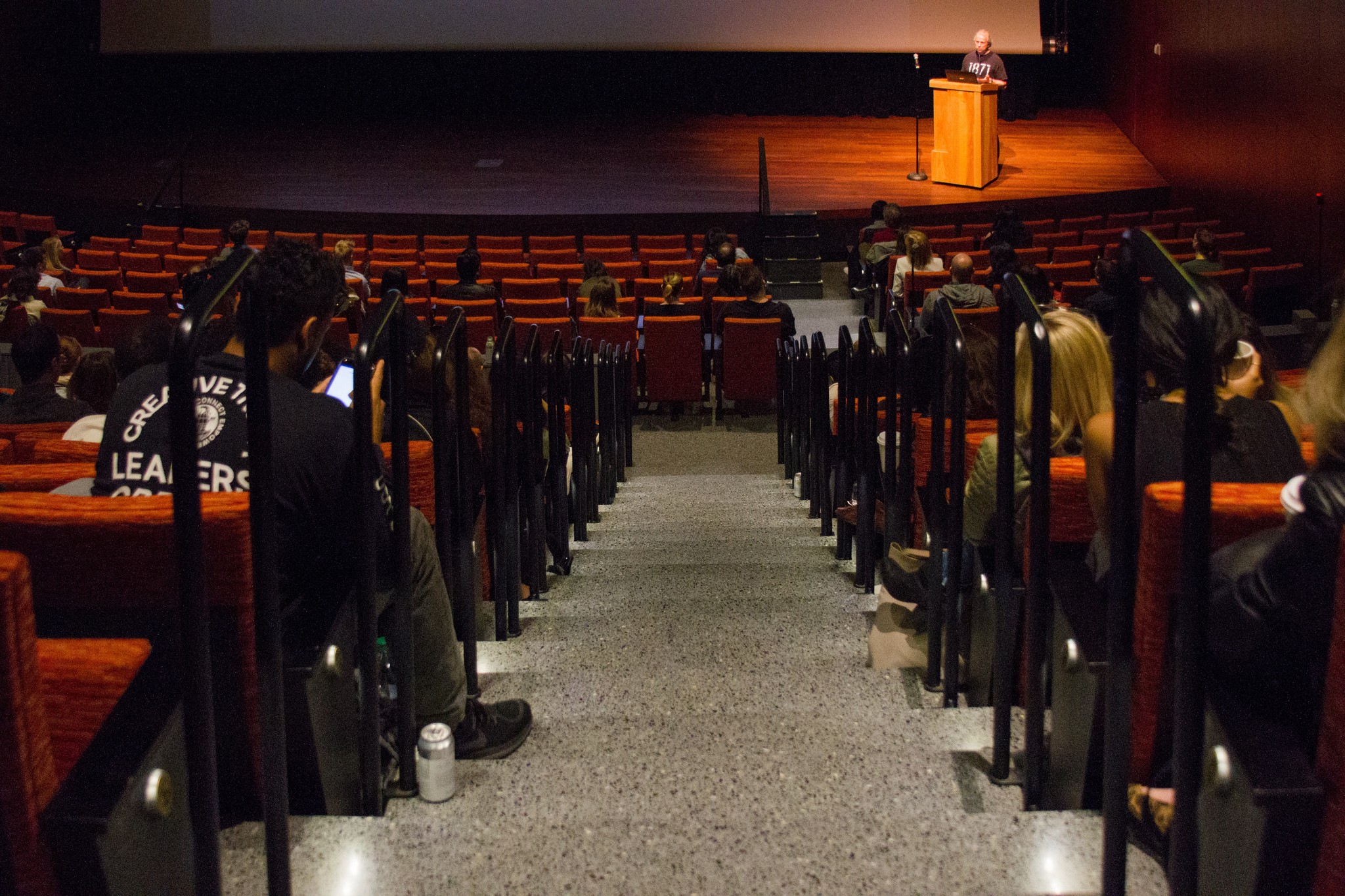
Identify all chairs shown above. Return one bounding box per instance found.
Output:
[0,208,1345,896]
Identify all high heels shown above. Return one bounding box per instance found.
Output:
[880,555,955,630]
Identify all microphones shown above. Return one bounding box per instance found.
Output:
[914,53,921,69]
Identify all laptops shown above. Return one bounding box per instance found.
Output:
[945,69,978,84]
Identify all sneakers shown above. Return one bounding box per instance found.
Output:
[452,695,534,760]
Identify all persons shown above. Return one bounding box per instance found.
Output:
[827,199,1345,870]
[0,220,624,761]
[642,226,797,351]
[961,29,1008,90]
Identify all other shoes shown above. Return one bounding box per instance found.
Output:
[835,498,885,532]
[1125,784,1173,840]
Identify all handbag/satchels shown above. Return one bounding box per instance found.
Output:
[866,534,995,683]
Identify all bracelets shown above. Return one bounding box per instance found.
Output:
[989,77,993,84]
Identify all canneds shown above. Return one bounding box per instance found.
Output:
[417,723,454,760]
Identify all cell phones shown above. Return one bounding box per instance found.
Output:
[324,357,360,408]
